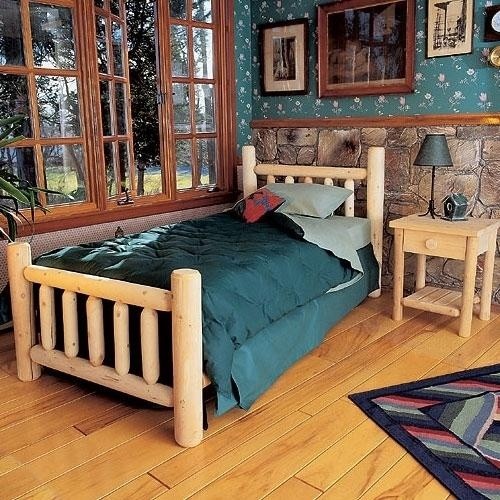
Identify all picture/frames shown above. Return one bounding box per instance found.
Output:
[258,17,310,96]
[316,0,418,99]
[425,0,474,59]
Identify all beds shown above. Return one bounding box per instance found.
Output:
[6,145,386,448]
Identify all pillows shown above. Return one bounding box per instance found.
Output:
[231,182,354,224]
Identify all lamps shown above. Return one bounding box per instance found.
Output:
[413,133,454,221]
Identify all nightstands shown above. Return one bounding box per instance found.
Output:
[389,212,500,339]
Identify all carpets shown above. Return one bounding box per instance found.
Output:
[348,361,500,497]
